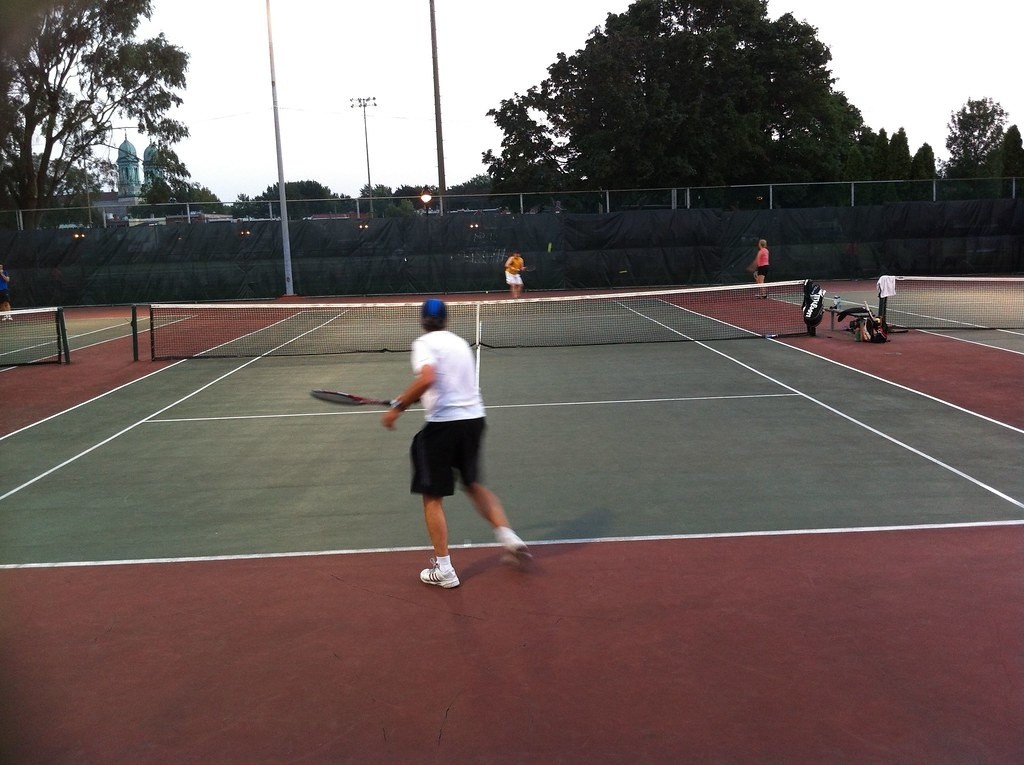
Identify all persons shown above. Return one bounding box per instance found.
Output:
[747,239,770,298]
[504,249,527,299]
[380,298,543,589]
[0,264,14,321]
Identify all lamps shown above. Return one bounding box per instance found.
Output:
[421,185,433,216]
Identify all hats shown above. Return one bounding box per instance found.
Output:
[422,297,447,320]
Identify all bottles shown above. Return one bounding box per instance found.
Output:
[854,326,861,342]
[834,296,841,311]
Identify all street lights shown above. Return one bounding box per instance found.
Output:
[419,185,432,291]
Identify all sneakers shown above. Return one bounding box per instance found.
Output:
[420,558,460,589]
[503,531,532,564]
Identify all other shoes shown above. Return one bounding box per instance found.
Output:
[1,312,14,322]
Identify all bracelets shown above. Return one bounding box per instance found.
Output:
[389,399,401,409]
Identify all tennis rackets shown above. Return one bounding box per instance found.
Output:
[309,388,422,407]
[525,264,536,271]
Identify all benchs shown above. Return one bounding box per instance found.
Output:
[824,307,869,332]
